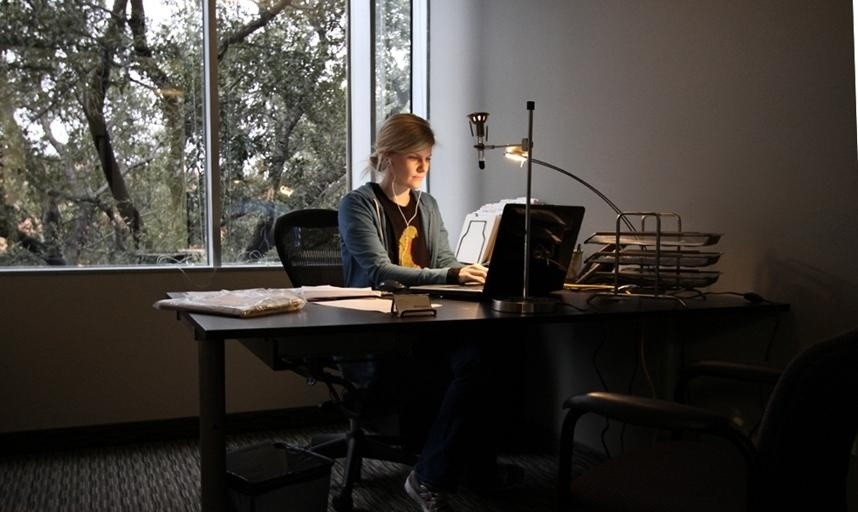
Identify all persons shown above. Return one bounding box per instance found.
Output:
[339,114,489,511]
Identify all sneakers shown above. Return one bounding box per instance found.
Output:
[405,472,443,511]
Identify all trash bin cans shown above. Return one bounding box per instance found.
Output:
[226,440,336,512]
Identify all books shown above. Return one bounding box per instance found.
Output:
[456,197,539,264]
[153,291,305,318]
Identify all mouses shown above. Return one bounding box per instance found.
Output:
[376,279,408,294]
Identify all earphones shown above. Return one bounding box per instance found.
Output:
[384,157,392,167]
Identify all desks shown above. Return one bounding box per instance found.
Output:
[166,285,789,512]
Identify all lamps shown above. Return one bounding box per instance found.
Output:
[504,143,660,295]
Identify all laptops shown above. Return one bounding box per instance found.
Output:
[409,204,585,304]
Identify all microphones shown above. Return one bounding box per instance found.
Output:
[476,124,486,170]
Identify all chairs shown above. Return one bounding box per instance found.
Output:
[272,207,419,511]
[556,323,858,512]
